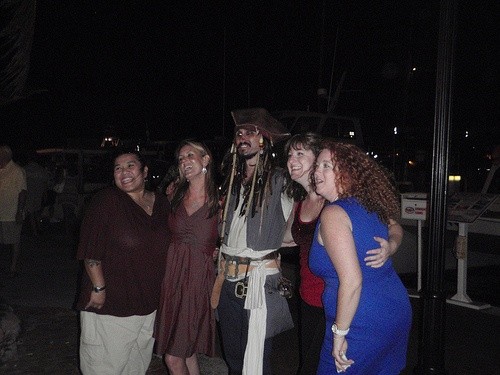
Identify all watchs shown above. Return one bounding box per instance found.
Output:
[92,285,106,293]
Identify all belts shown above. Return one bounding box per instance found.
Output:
[222,251,279,265]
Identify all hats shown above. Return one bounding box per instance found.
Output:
[234,109,291,144]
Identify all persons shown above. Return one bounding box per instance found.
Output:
[282,130,405,374]
[152,139,223,375]
[307,142,415,374]
[212,107,301,374]
[76,148,172,375]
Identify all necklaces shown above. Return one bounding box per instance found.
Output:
[186,191,205,210]
[132,198,156,211]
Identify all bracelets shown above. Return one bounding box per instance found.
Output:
[331,322,350,336]
